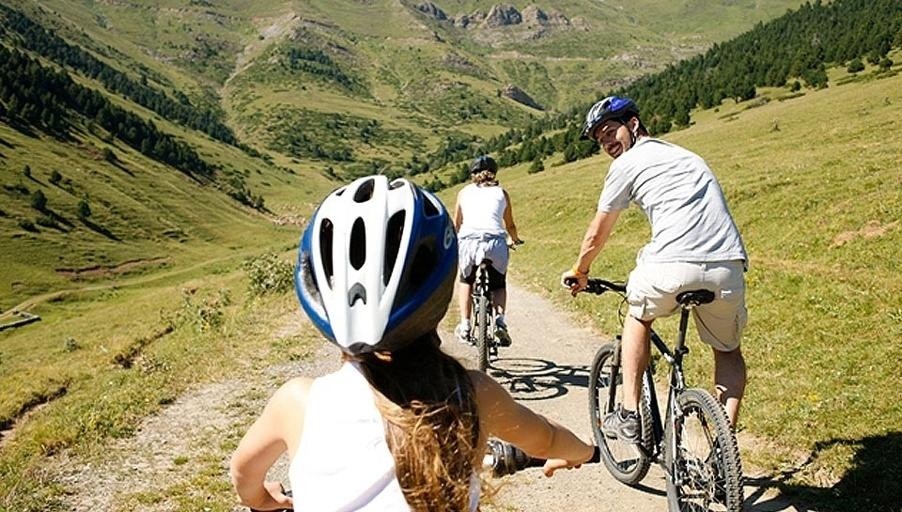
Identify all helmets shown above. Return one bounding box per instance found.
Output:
[578,96,639,139]
[294,174,459,361]
[470,155,498,175]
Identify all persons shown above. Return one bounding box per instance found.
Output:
[227,174,595,511]
[453,155,520,347]
[560,88,750,494]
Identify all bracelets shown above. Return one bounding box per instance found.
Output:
[572,264,590,277]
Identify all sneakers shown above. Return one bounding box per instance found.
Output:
[493,321,512,346]
[455,324,472,342]
[706,437,724,471]
[602,404,642,444]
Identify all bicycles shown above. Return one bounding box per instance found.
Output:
[464,240,526,373]
[565,276,746,512]
